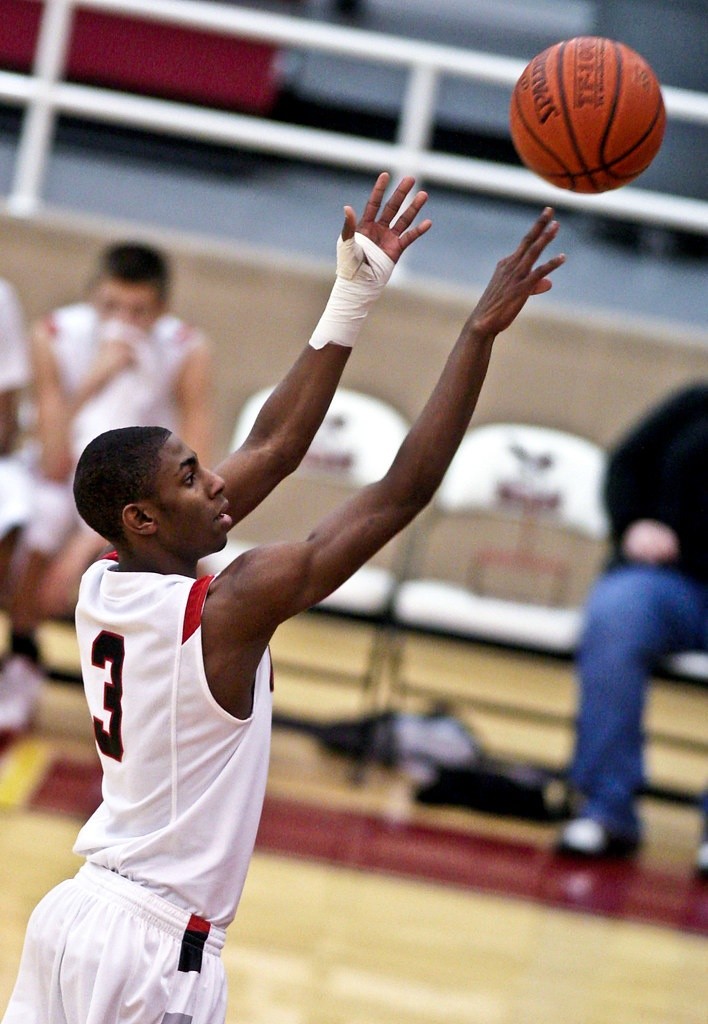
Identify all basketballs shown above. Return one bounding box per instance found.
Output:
[509,34,668,195]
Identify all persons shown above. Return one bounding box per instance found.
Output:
[0,171,567,1024]
[557,379,708,874]
[0,238,212,735]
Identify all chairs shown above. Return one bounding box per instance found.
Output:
[636,649,708,807]
[382,420,609,790]
[201,386,416,785]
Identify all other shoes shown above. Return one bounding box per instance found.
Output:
[555,817,639,858]
[0,655,43,731]
